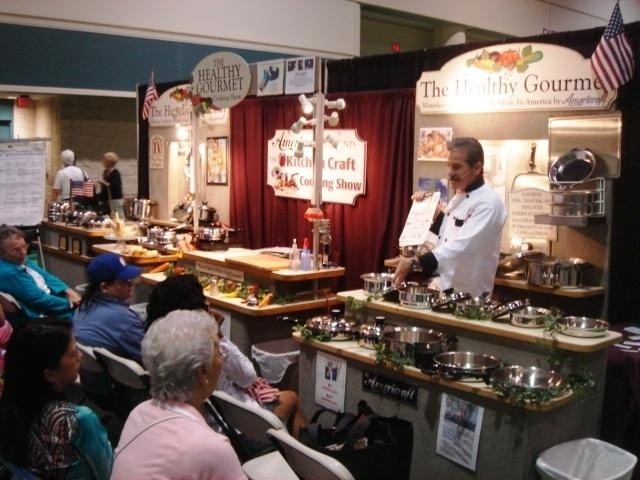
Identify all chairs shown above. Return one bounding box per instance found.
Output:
[75,342,106,408]
[268,428,359,480]
[1,289,21,321]
[94,340,150,419]
[202,387,288,463]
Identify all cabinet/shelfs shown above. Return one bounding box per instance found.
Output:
[30,214,138,298]
[291,289,623,480]
[141,246,347,376]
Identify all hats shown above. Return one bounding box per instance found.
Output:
[87,254,143,285]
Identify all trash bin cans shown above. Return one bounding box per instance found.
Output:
[536,437,637,480]
[252,337,300,395]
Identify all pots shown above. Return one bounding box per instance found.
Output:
[512,307,551,328]
[398,285,441,310]
[146,227,175,244]
[306,314,403,350]
[522,254,588,287]
[375,325,452,368]
[547,188,595,217]
[453,291,498,315]
[187,228,227,242]
[197,201,215,223]
[123,198,157,221]
[172,192,195,223]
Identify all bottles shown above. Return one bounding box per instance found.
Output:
[289,238,312,271]
[208,276,217,295]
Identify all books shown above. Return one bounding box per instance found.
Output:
[398,190,442,248]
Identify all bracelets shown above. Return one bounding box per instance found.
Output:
[410,259,419,274]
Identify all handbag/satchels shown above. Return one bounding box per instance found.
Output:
[299,409,414,478]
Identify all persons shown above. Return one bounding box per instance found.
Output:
[51,149,89,203]
[2,317,116,479]
[111,308,251,479]
[67,252,150,374]
[0,223,82,323]
[383,136,510,304]
[141,273,310,449]
[1,303,13,398]
[96,152,124,213]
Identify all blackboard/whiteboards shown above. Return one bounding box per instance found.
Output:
[509,191,556,242]
[3,142,47,227]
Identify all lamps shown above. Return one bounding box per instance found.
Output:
[289,94,345,206]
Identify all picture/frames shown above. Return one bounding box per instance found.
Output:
[205,135,228,187]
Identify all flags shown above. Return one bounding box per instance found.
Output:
[70,181,95,199]
[589,4,637,95]
[141,76,159,121]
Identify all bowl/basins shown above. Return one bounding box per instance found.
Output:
[359,273,396,296]
[433,350,502,384]
[496,362,563,392]
[547,149,596,186]
[555,316,610,337]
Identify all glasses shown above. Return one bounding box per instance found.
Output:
[213,344,229,358]
